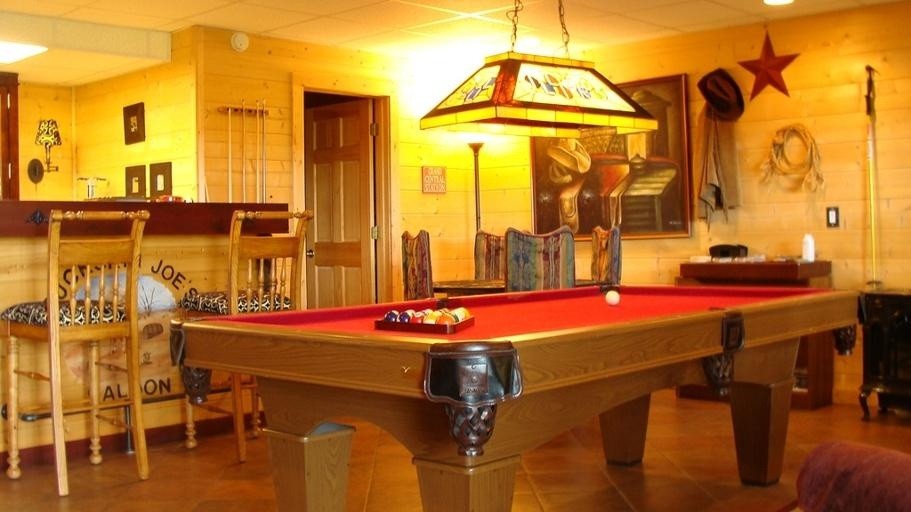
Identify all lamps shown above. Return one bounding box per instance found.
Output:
[420,0,659,138]
[28,120,61,184]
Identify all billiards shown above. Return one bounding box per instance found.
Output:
[605,290,620,306]
[385,307,471,325]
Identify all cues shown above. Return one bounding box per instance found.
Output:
[241,98,246,202]
[227,104,233,204]
[255,97,262,204]
[261,98,267,203]
[865,68,877,283]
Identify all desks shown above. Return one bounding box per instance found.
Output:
[675,260,832,410]
[860,288,911,422]
[433,279,593,297]
[171,284,867,512]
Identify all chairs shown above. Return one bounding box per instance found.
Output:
[1,209,151,497]
[503,225,576,292]
[474,230,531,280]
[401,229,433,301]
[176,209,315,463]
[591,227,622,285]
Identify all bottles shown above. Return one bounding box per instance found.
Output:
[802,234,815,264]
[87,181,95,199]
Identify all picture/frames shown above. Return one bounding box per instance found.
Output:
[531,72,694,242]
[150,162,172,198]
[125,165,146,199]
[123,102,145,145]
